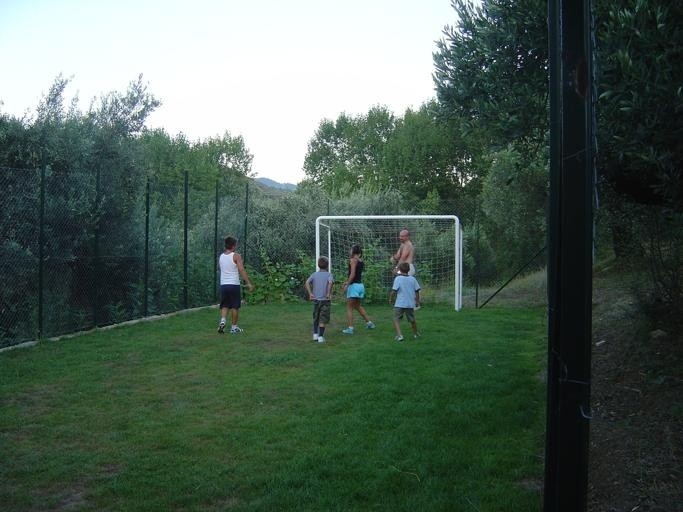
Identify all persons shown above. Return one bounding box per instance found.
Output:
[389,262,421,342]
[304,256,334,345]
[340,245,376,334]
[217,235,253,334]
[389,229,421,311]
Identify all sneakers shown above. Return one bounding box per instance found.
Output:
[412,332,421,338]
[342,327,354,335]
[414,305,422,310]
[364,321,376,329]
[317,336,327,345]
[218,320,226,334]
[394,335,404,341]
[313,333,320,341]
[230,326,244,334]
